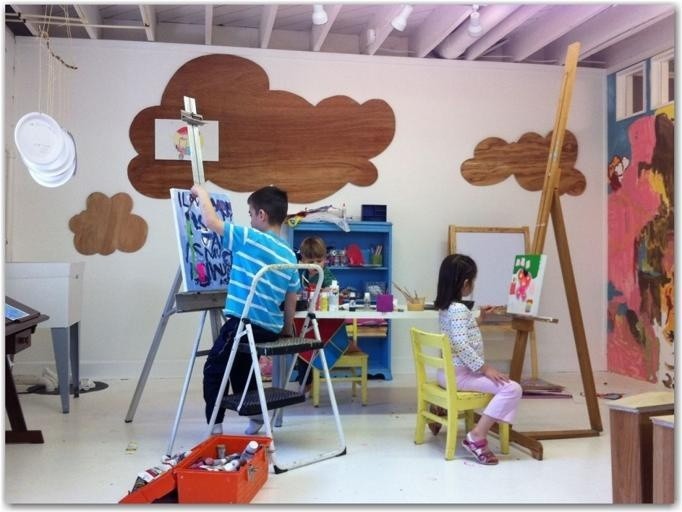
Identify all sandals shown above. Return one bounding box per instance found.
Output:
[428,404,443,435]
[462,432,498,465]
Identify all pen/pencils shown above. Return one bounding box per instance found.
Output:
[480,305,507,310]
[393,283,418,304]
[368,244,383,255]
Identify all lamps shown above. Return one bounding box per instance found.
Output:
[311,3,330,24]
[468,5,485,35]
[390,5,415,31]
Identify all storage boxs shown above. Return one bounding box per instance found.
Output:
[117,433,273,504]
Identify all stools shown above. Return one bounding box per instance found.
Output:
[202,264,347,475]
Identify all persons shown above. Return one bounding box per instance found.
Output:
[190,184,301,438]
[295,236,340,394]
[428,255,523,465]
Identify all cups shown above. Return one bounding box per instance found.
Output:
[407,297,425,311]
[369,254,384,267]
[296,295,307,311]
[376,294,394,312]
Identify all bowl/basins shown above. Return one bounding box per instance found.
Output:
[461,301,475,310]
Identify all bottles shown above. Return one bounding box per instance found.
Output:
[304,279,340,311]
[349,292,356,312]
[342,203,347,222]
[396,293,405,313]
[363,293,371,312]
[201,440,259,472]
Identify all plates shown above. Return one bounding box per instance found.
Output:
[14,112,77,188]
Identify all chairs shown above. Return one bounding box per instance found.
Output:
[410,325,510,460]
[312,318,368,408]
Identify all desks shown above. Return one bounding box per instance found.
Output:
[273,306,478,428]
[5,262,85,413]
